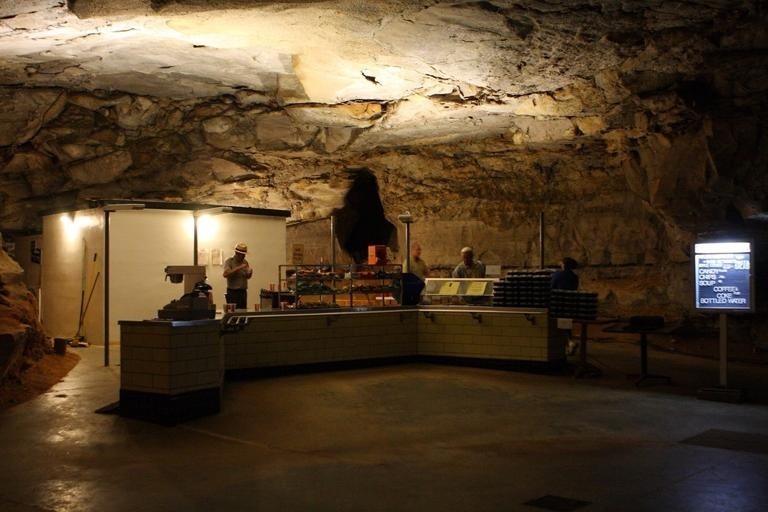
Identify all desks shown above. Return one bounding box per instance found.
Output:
[564,318,695,385]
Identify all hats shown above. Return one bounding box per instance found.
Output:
[233,243,248,255]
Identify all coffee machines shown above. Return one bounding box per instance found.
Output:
[158,265,214,319]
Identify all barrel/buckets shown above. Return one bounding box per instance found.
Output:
[54,338,67,355]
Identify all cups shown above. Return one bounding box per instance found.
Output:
[208,303,216,319]
[223,303,237,313]
[254,304,260,311]
[269,282,276,291]
[280,302,289,311]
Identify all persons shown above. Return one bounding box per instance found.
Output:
[552,256,581,357]
[401,241,432,305]
[222,242,254,309]
[450,246,491,306]
